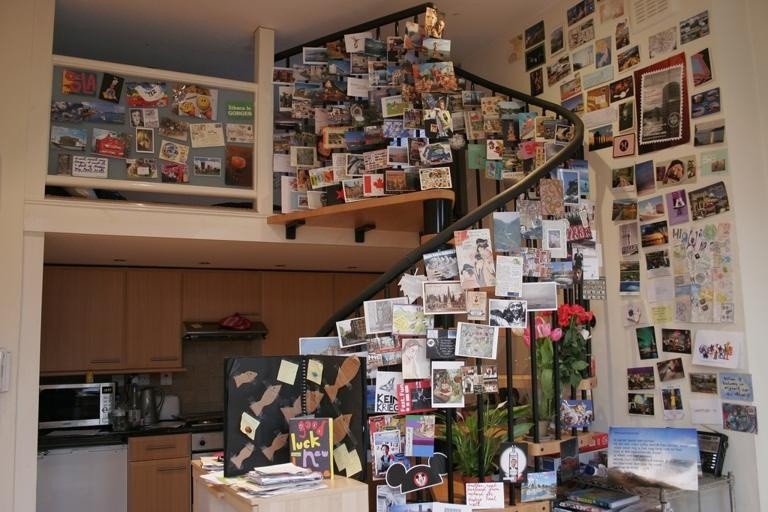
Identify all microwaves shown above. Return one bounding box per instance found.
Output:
[38,381,116,432]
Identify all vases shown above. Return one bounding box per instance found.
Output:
[522,417,556,442]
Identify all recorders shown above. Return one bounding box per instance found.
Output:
[697,431,729,477]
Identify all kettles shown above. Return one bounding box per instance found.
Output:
[140,385,165,426]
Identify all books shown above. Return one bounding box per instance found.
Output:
[551,486,641,512]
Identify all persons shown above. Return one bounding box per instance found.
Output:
[424,7,438,36]
[460,238,496,288]
[574,247,584,269]
[316,134,334,161]
[503,301,526,328]
[667,359,684,380]
[432,16,448,39]
[322,79,339,94]
[509,459,518,471]
[273,68,296,84]
[435,96,453,138]
[402,339,423,379]
[380,444,392,472]
[520,224,530,239]
[129,108,144,128]
[573,268,583,284]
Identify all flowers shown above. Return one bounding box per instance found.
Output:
[511,304,597,418]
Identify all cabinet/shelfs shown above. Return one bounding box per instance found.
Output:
[569,464,735,512]
[127,271,184,373]
[335,270,388,322]
[388,272,399,298]
[261,272,335,357]
[127,433,224,512]
[182,269,262,323]
[43,264,125,373]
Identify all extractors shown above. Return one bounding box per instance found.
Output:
[180,316,269,344]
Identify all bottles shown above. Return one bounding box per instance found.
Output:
[112,408,127,432]
[128,382,141,427]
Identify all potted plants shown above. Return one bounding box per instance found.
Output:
[430,400,536,502]
